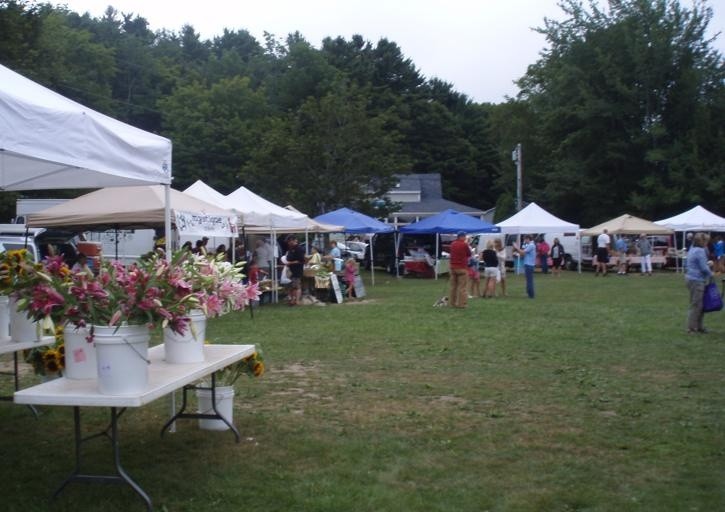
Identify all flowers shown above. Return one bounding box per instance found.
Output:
[32,252,264,342]
[202,342,264,386]
[0,248,38,296]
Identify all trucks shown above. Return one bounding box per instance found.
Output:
[5,198,231,269]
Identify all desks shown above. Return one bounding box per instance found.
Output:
[0,335,57,422]
[592,254,667,273]
[13,343,256,512]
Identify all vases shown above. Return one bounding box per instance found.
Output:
[1,297,12,341]
[8,298,39,344]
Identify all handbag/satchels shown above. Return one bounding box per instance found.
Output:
[701,277,723,312]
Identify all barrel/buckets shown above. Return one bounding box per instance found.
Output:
[10,298,43,341]
[0,296,10,341]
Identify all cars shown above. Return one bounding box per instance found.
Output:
[338,240,369,262]
[299,240,349,258]
[636,235,672,264]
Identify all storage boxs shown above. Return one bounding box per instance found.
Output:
[302,264,321,276]
[314,275,330,289]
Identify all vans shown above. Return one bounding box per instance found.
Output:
[0,222,48,265]
[362,230,592,269]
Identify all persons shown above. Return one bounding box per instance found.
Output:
[538,238,550,273]
[550,238,564,271]
[596,230,653,276]
[182,234,357,306]
[512,235,536,298]
[448,230,506,308]
[684,232,725,333]
[54,244,95,279]
